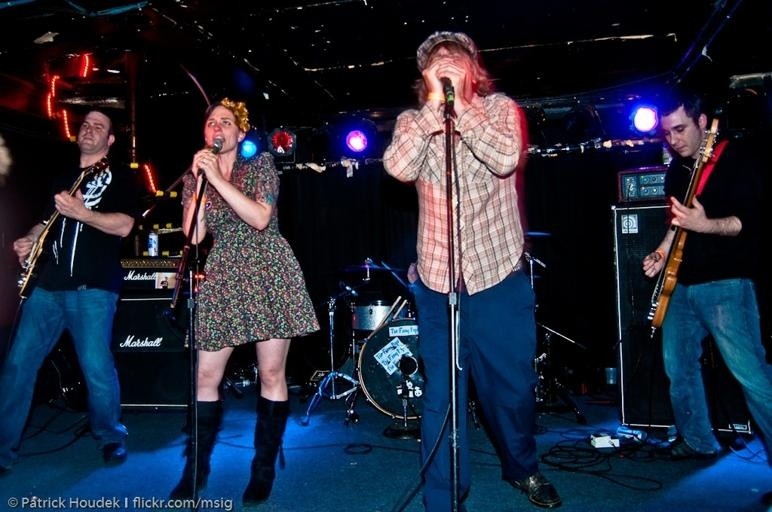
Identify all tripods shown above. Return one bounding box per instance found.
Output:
[301,325,360,426]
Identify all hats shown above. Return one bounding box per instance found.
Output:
[415,32,478,67]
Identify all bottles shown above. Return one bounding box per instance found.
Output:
[148,232,159,256]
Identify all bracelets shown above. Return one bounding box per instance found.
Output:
[655,246,668,265]
[427,91,445,105]
[192,192,207,205]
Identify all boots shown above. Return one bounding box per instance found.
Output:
[244,396,291,506]
[169,400,225,506]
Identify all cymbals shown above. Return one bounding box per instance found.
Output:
[349,264,403,273]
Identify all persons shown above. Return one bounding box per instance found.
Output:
[383,29,565,512]
[169,98,320,506]
[0,109,143,477]
[643,86,772,511]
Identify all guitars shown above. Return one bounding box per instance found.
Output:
[18,156,108,298]
[648,118,719,327]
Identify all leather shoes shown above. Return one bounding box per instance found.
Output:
[663,437,720,460]
[510,473,564,509]
[100,448,129,464]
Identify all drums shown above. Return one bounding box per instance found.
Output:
[357,317,425,420]
[353,299,406,331]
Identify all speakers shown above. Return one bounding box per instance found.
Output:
[612,204,755,437]
[111,269,199,410]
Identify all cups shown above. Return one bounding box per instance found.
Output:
[605,367,616,384]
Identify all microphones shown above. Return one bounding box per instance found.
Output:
[442,78,455,106]
[196,140,221,176]
